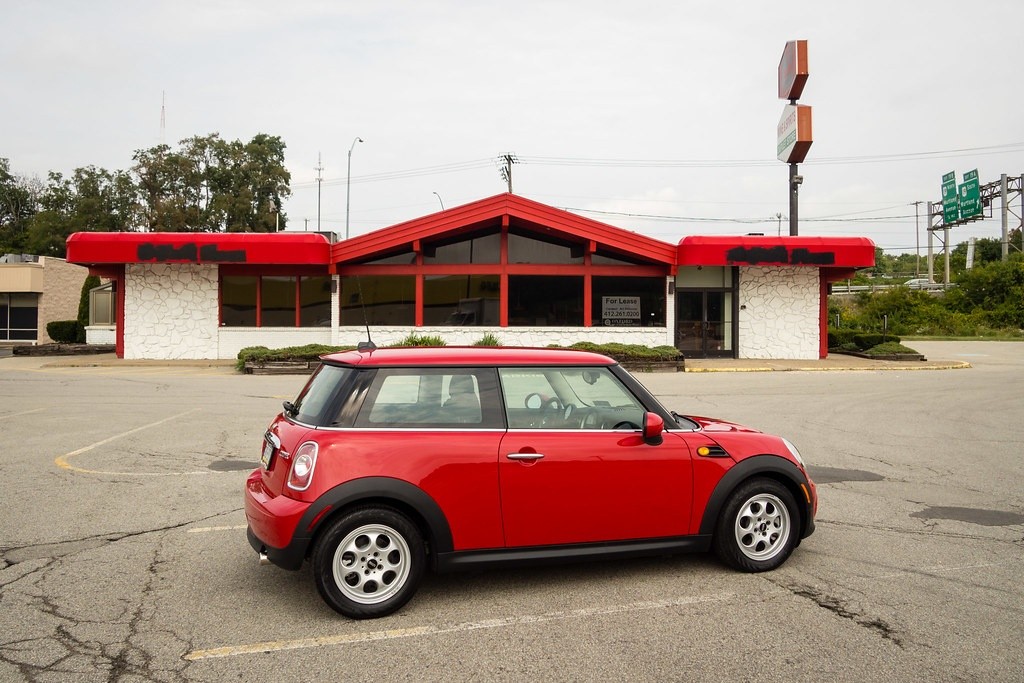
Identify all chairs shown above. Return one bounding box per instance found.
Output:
[444,373,480,423]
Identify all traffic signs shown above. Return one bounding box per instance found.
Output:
[955,168,983,218]
[939,170,960,224]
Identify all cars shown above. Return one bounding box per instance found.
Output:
[903,277,938,291]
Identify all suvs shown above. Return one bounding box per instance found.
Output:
[243,341,819,621]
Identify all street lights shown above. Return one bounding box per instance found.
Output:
[345,137,364,240]
